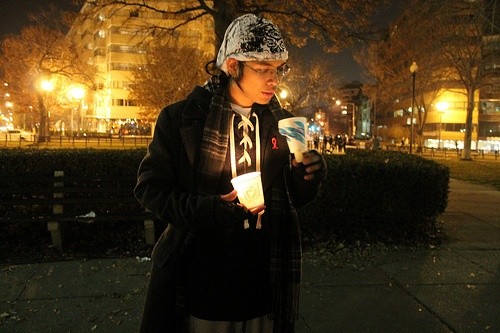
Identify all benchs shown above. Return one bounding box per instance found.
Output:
[0,171,155,249]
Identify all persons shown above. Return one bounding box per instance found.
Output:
[134,15,327,333]
[308,133,355,155]
[369,134,423,158]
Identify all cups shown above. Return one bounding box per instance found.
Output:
[231,171,265,215]
[278,116,310,165]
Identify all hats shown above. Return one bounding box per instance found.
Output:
[216,14,288,77]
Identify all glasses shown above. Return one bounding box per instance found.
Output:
[241,61,292,78]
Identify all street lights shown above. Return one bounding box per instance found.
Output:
[409,61,419,155]
[40,79,53,142]
[279,89,287,108]
[72,87,86,140]
[436,102,447,150]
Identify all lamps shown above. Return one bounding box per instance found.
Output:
[230,172,264,213]
